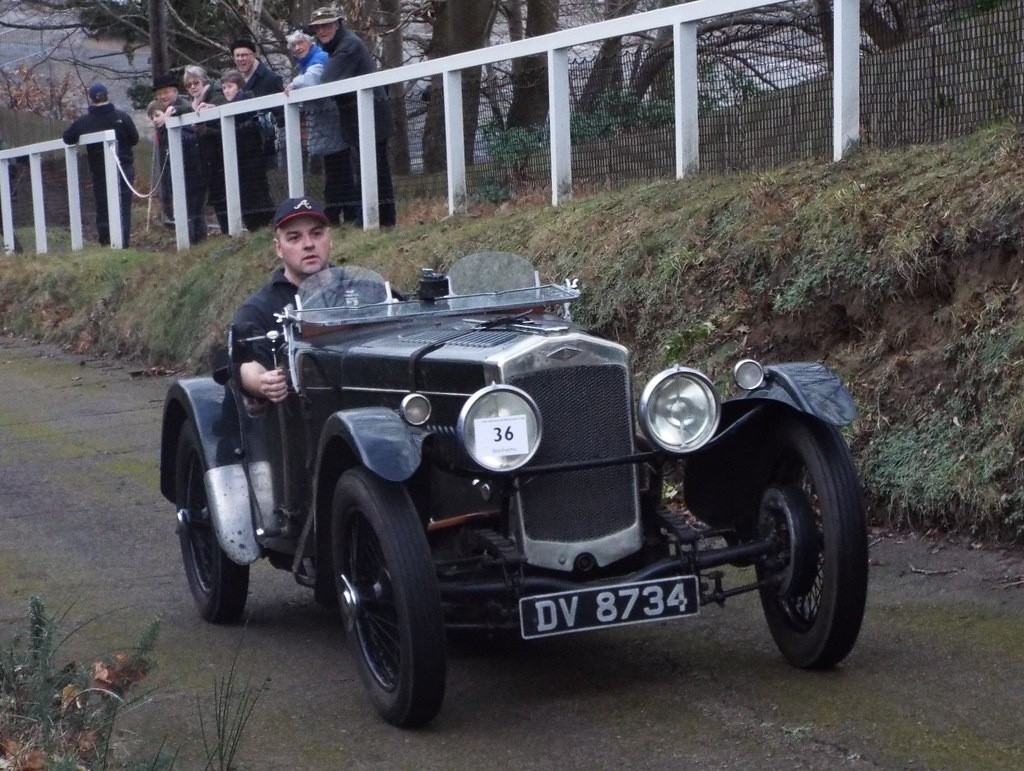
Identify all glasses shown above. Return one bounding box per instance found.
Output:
[185,81,201,87]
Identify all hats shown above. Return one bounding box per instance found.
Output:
[88,84,106,101]
[151,76,178,89]
[230,39,256,52]
[308,7,346,25]
[272,197,330,229]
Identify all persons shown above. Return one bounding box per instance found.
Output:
[285,30,351,229]
[230,39,285,170]
[308,7,396,226]
[232,197,404,404]
[183,64,229,234]
[146,74,209,245]
[62,83,139,248]
[222,70,276,232]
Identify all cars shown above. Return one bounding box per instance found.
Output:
[159,253,868,730]
